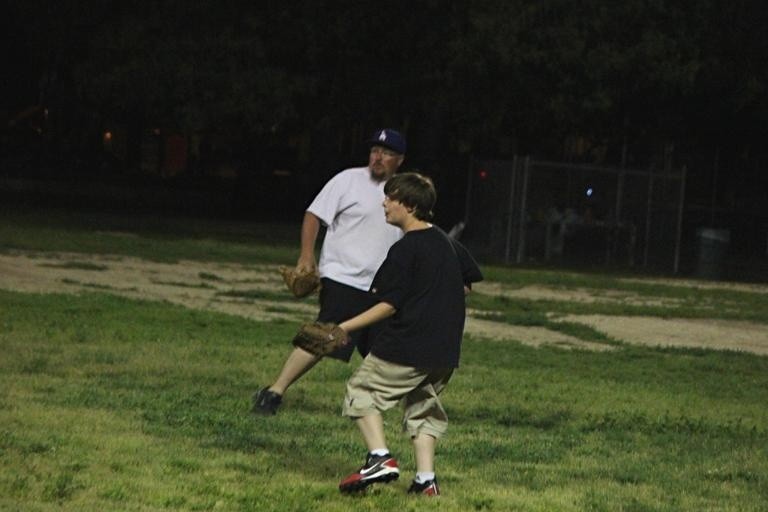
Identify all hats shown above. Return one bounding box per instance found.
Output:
[372,127,407,154]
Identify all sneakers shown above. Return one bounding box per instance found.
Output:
[340,452,400,492]
[406,475,440,497]
[251,386,282,416]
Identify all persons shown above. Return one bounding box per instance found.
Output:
[290,167,484,496]
[248,128,420,417]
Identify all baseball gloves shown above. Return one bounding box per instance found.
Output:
[279,265,319,300]
[292,322,355,362]
